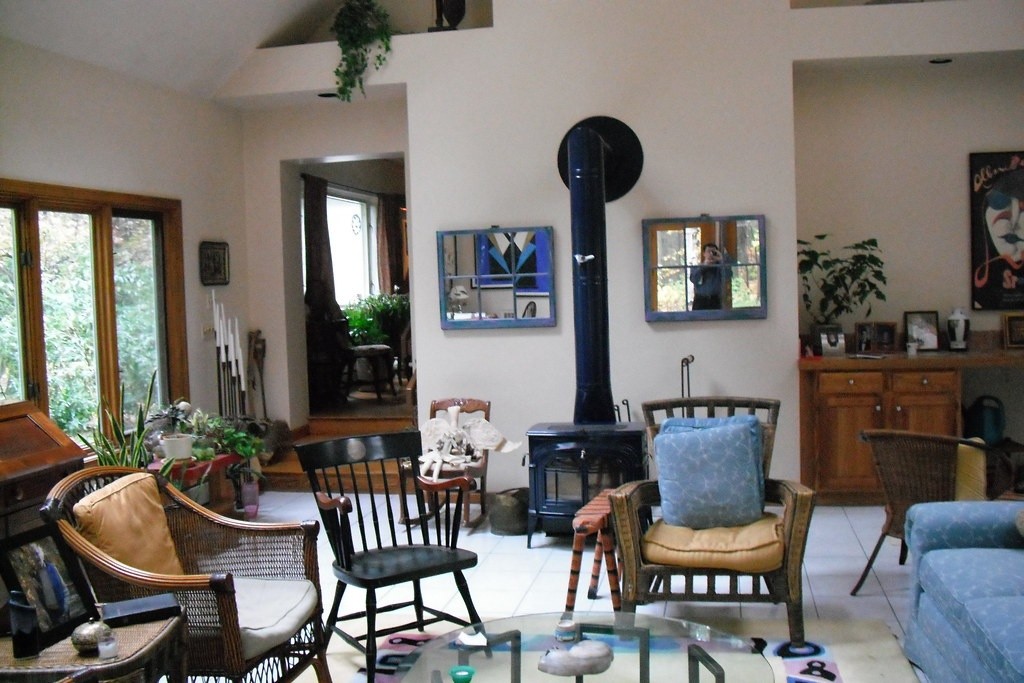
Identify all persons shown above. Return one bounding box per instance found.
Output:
[860,328,871,350]
[690,243,733,310]
[28,544,70,631]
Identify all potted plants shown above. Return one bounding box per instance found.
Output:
[797,233,887,355]
[77,369,266,521]
[330,0,391,103]
[341,292,410,383]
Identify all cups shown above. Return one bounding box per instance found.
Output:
[449,665,475,683]
[906,341,919,357]
[97,630,119,663]
[158,433,197,461]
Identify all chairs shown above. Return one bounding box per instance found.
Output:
[323,296,398,407]
[396,396,494,529]
[293,430,494,683]
[849,429,1024,596]
[38,465,333,683]
[603,395,817,648]
[522,301,536,318]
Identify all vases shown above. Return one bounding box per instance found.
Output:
[945,305,972,353]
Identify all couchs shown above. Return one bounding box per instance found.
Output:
[900,498,1024,683]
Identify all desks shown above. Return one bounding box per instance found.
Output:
[393,609,777,683]
[0,599,190,683]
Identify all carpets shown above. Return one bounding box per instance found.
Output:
[343,630,846,683]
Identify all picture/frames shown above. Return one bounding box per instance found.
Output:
[199,240,231,286]
[470,229,551,297]
[904,310,942,353]
[1001,310,1024,351]
[967,147,1024,311]
[854,321,898,354]
[0,524,104,653]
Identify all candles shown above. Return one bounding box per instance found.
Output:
[211,288,247,393]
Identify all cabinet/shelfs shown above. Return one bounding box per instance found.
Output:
[0,401,87,532]
[798,349,1024,513]
[525,422,653,549]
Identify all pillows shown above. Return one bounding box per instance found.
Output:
[908,435,988,502]
[653,413,767,531]
[71,471,186,600]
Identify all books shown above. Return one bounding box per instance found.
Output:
[102,592,181,628]
[857,351,885,360]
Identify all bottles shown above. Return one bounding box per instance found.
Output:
[240,475,259,520]
[945,307,971,350]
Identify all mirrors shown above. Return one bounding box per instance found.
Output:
[436,224,557,331]
[641,214,767,323]
